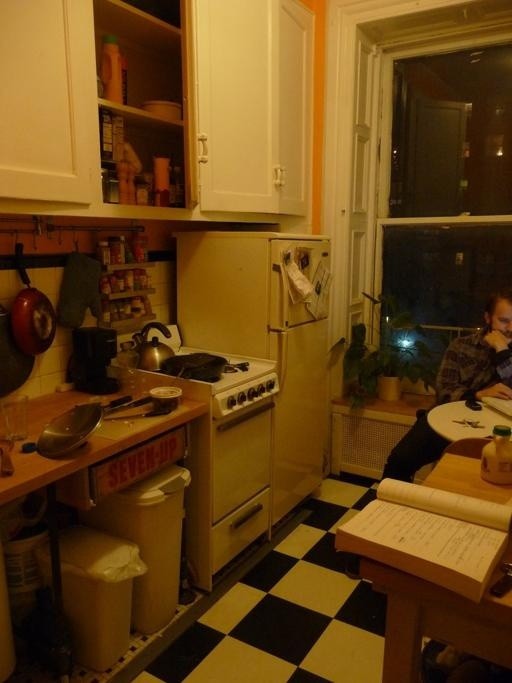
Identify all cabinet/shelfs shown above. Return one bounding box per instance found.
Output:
[193,1,317,217]
[97,1,210,213]
[0,0,92,208]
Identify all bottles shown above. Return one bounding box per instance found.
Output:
[118,341,139,368]
[481,425,512,485]
[98,224,153,323]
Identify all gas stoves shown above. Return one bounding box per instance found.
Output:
[205,354,249,383]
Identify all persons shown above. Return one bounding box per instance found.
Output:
[381,286,512,484]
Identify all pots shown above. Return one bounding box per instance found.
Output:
[161,353,227,380]
[11,243,57,357]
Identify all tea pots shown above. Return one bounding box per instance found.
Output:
[132,322,174,371]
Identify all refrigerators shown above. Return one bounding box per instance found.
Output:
[177,230,331,527]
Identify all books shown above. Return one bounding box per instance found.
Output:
[481,393,512,418]
[333,475,512,607]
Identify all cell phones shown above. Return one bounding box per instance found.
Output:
[465,400,482,411]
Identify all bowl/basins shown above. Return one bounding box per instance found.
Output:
[150,387,182,410]
[144,100,182,120]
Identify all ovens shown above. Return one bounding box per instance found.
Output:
[210,373,279,576]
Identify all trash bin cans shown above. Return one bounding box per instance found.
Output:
[61,466,191,673]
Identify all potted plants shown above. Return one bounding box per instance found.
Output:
[325,286,450,412]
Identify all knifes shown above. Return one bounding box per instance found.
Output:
[103,396,171,416]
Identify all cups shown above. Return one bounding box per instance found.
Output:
[2,393,27,440]
